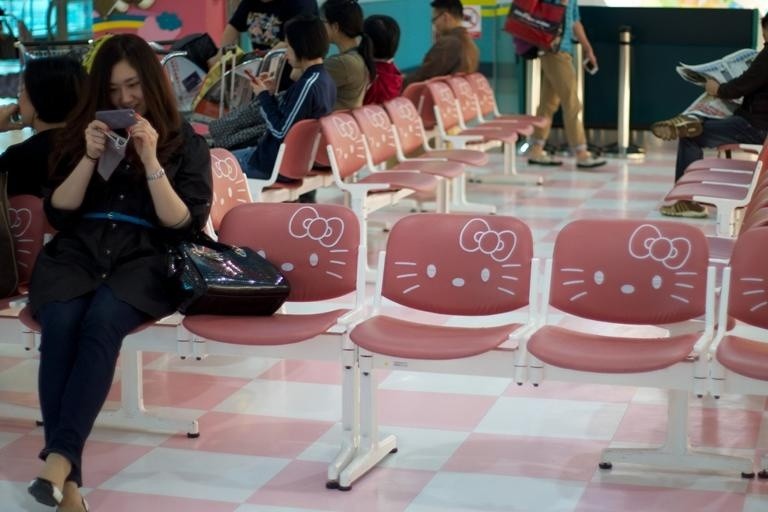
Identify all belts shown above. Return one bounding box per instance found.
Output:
[81,210,154,230]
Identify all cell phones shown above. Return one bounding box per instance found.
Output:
[244,67,257,83]
[95,108,137,130]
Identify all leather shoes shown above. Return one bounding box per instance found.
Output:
[56,495,88,511]
[27,476,64,507]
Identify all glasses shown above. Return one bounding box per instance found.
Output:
[430,11,446,24]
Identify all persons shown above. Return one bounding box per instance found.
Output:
[364,15,405,107]
[648,12,768,218]
[525,0,607,170]
[230,12,338,181]
[0,58,81,200]
[26,32,215,512]
[205,1,376,151]
[398,0,480,91]
[220,1,320,57]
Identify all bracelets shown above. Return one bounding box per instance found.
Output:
[85,152,101,162]
[145,167,165,181]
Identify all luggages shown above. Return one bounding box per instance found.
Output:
[202,44,237,149]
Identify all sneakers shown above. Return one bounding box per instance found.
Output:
[660,200,709,218]
[576,153,606,168]
[528,154,563,165]
[651,114,703,141]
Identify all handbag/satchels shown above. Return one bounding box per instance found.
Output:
[504,0,566,55]
[164,242,290,317]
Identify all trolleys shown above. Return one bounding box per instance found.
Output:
[13,38,96,99]
[145,33,287,143]
